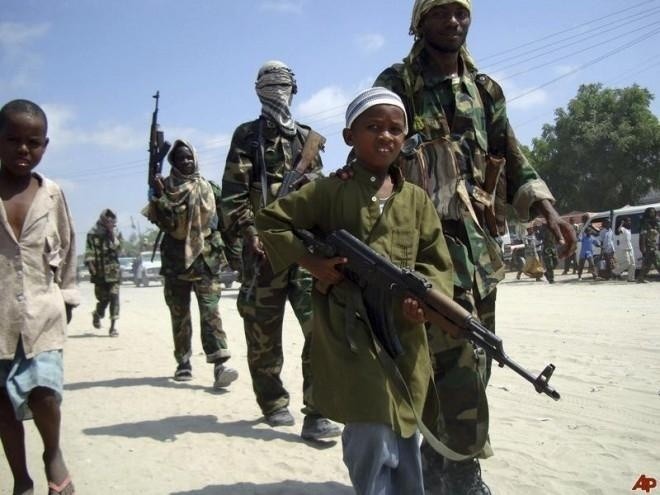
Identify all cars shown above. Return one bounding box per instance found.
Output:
[118,258,136,282]
[132,251,164,287]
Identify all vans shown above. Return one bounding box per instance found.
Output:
[612,204,659,269]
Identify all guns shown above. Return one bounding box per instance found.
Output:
[247,132,328,296]
[291,228,562,401]
[149,90,178,234]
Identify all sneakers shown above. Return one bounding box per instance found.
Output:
[108,327,119,338]
[92,311,102,329]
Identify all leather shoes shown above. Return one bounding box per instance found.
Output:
[421,448,444,494]
[445,458,491,495]
[298,416,345,441]
[210,365,239,393]
[265,405,296,427]
[174,364,192,381]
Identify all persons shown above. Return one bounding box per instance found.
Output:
[84,210,123,337]
[219,60,344,439]
[540,224,558,283]
[637,207,660,283]
[254,87,455,495]
[598,221,615,281]
[141,140,239,391]
[562,224,580,274]
[577,226,601,281]
[517,228,543,281]
[331,0,577,494]
[614,216,636,282]
[0,99,80,495]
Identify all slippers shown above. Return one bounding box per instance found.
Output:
[46,474,76,495]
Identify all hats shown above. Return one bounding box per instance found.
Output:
[344,83,409,135]
